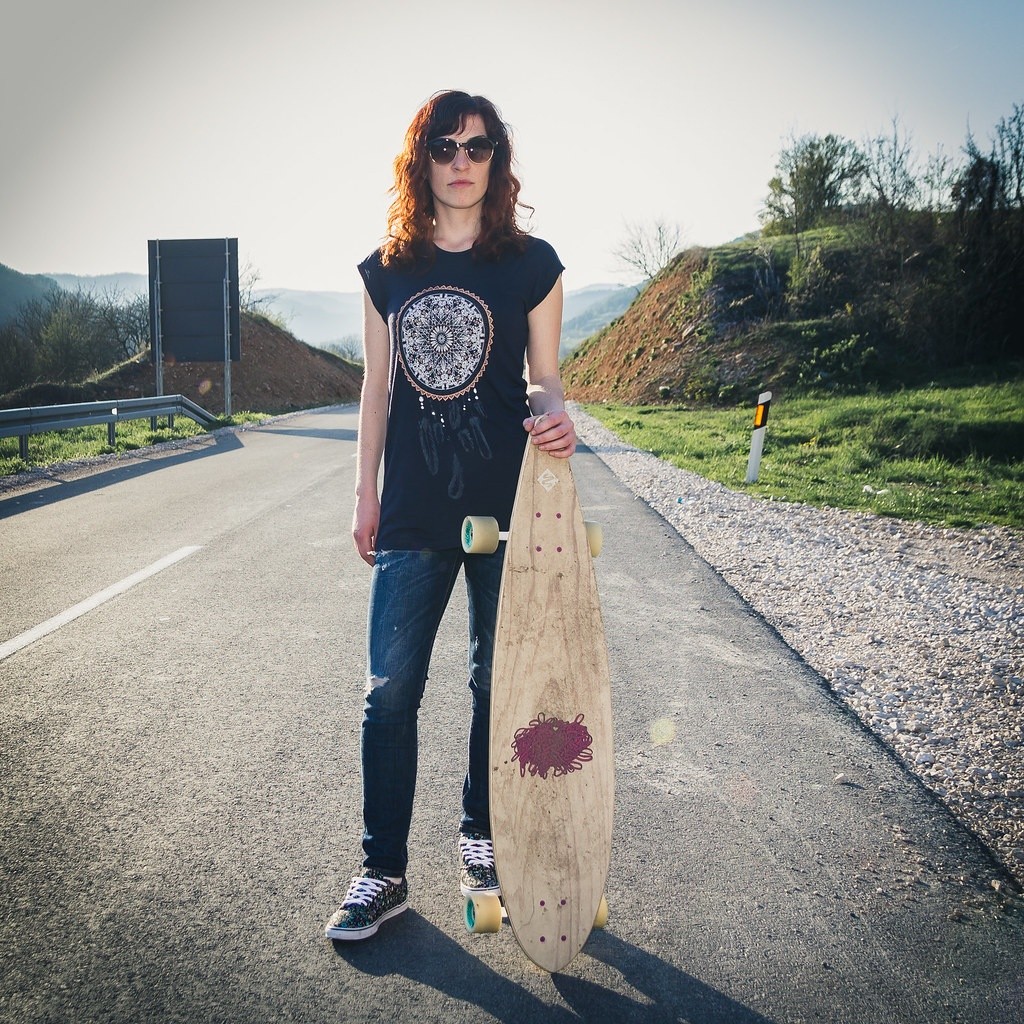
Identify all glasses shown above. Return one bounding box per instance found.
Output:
[428,136,498,165]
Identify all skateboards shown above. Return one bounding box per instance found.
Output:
[460,411,625,978]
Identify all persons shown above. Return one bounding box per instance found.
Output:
[325,90,576,941]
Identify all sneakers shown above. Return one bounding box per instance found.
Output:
[458,828,501,898]
[324,866,409,940]
